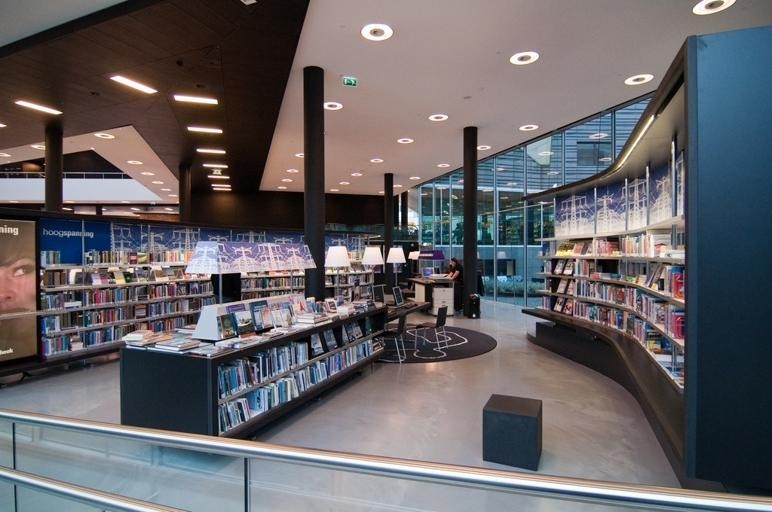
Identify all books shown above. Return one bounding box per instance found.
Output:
[241,269,305,300]
[325,251,373,302]
[191,296,373,431]
[552,231,684,390]
[41,249,217,356]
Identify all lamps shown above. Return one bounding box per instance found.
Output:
[182,238,316,340]
[327,243,408,305]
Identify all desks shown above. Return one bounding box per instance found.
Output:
[408,276,455,317]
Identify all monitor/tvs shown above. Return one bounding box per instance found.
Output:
[422,267,434,278]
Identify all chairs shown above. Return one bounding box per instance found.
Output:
[375,306,453,366]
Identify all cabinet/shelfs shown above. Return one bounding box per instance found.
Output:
[539,215,688,382]
[35,261,389,437]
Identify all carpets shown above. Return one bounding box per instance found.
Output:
[385,320,498,364]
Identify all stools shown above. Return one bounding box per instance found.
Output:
[481,392,544,471]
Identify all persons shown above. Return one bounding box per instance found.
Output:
[446,257,464,316]
[0,219,38,362]
